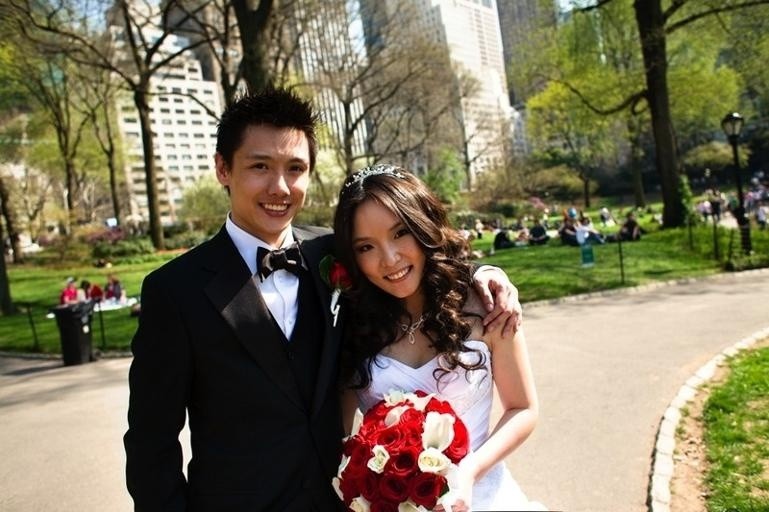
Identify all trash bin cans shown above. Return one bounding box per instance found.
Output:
[48,302,100,366]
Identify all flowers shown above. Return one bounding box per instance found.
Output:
[329,388,472,512]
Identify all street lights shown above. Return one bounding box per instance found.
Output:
[722,110,751,252]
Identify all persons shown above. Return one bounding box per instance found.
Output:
[330,159,551,511]
[696,172,769,255]
[121,78,522,511]
[61,276,83,305]
[459,203,641,251]
[79,279,103,302]
[97,275,126,307]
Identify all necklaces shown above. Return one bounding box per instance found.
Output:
[398,308,432,346]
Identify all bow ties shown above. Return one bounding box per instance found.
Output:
[256,248,307,282]
